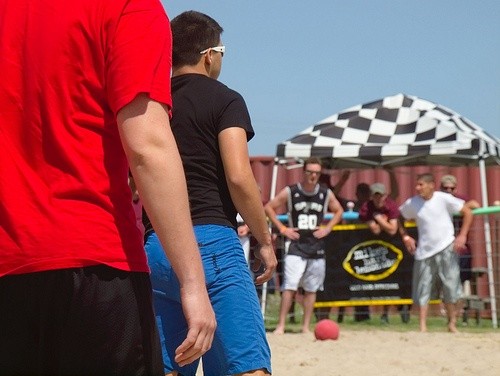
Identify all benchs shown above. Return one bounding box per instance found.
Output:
[309,299,443,306]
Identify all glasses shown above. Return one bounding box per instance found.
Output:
[199,45,224,58]
[443,186,455,191]
[304,170,322,177]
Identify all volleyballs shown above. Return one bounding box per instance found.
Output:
[314,319,338,340]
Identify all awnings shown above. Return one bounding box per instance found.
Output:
[259,91,494,335]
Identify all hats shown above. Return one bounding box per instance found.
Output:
[441,174,457,184]
[368,183,386,196]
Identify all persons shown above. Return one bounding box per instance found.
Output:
[130,10,272,376]
[442,174,479,293]
[320,170,400,238]
[396,172,472,331]
[266,158,342,335]
[0,0,218,376]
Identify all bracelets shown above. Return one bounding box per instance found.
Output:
[278,225,288,234]
